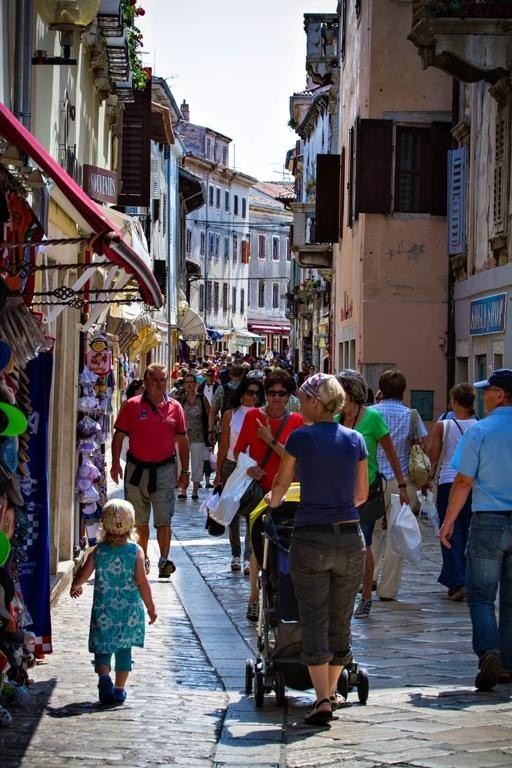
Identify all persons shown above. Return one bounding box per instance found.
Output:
[110,363,191,579]
[333,368,410,618]
[167,347,300,625]
[437,368,512,690]
[264,372,370,725]
[427,392,481,457]
[120,378,146,463]
[298,361,309,388]
[321,348,329,374]
[359,369,434,601]
[304,364,316,381]
[69,498,158,706]
[84,334,113,398]
[429,382,478,602]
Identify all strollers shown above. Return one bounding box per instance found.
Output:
[245,481,370,706]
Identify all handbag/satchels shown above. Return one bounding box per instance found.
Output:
[207,450,258,527]
[416,489,439,532]
[238,407,289,516]
[388,492,422,571]
[209,451,218,471]
[409,408,430,487]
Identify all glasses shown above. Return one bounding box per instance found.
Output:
[267,390,288,397]
[184,381,193,383]
[245,390,260,396]
[207,374,213,377]
[483,388,502,392]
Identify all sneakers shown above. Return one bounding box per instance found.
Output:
[144,558,150,574]
[178,494,188,501]
[98,676,114,703]
[192,496,198,500]
[113,687,126,701]
[245,601,259,621]
[158,558,176,578]
[355,595,372,616]
[359,581,377,593]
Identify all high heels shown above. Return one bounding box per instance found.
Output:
[448,586,466,600]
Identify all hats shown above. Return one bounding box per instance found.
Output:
[473,369,512,390]
[299,372,345,412]
[102,498,135,535]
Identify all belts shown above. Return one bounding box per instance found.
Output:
[295,522,359,535]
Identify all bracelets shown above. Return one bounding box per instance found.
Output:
[397,483,407,488]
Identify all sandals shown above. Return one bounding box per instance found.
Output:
[198,485,202,488]
[475,652,503,690]
[243,560,250,575]
[498,670,511,683]
[313,696,336,710]
[206,484,213,488]
[231,557,241,571]
[304,699,332,724]
